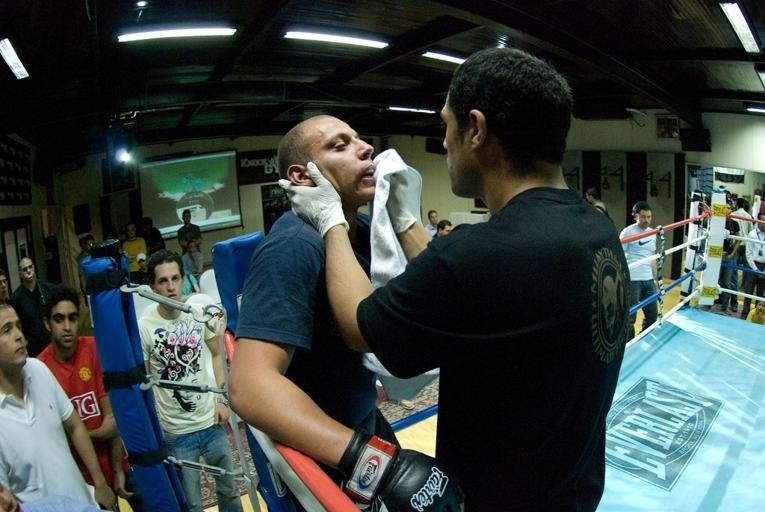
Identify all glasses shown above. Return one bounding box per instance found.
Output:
[138,260,145,263]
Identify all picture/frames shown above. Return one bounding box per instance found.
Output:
[655,113,679,141]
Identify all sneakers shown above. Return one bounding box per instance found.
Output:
[732,306,738,312]
[741,310,748,319]
[714,299,723,304]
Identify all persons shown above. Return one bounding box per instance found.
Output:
[425,209,439,237]
[586,189,609,217]
[276,48,632,512]
[0,301,121,511]
[12,255,53,358]
[227,113,470,509]
[618,202,659,340]
[0,478,25,512]
[35,283,138,512]
[709,192,765,323]
[433,220,452,241]
[0,209,204,310]
[136,250,243,511]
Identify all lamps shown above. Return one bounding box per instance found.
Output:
[744,106,765,115]
[117,27,237,44]
[387,106,437,113]
[420,50,468,65]
[718,2,762,54]
[281,25,391,49]
[0,37,31,80]
[754,63,765,89]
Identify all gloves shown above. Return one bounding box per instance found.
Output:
[278,162,351,238]
[336,427,465,512]
[386,172,417,234]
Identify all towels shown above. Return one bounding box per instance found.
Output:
[362,147,441,405]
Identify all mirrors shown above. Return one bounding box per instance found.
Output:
[685,162,714,237]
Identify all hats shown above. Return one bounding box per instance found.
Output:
[136,253,146,262]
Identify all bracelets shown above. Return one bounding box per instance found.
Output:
[218,398,230,407]
